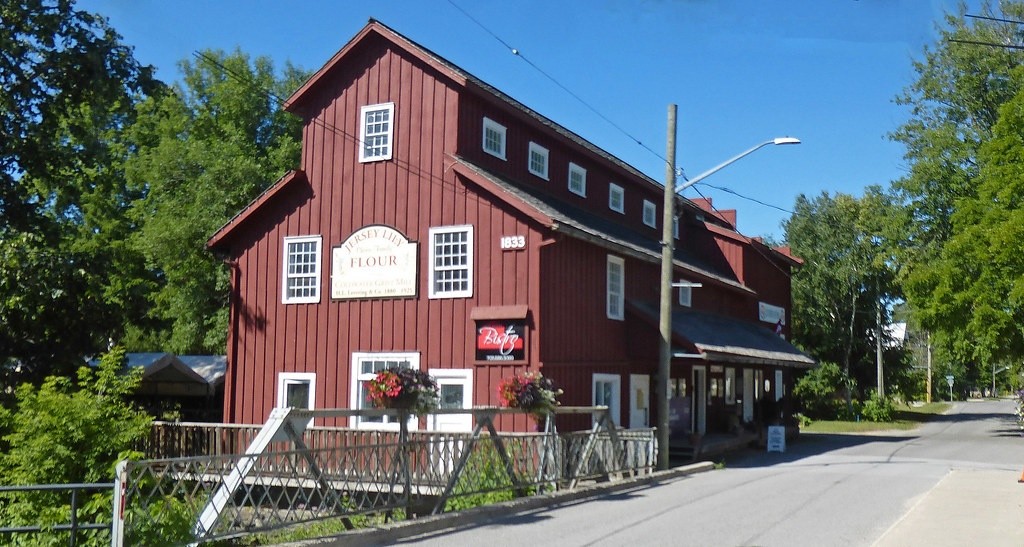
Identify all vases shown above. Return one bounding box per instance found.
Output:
[384,398,415,410]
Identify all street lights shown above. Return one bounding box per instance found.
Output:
[655,104,801,474]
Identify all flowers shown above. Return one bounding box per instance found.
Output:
[496,369,563,424]
[362,366,439,409]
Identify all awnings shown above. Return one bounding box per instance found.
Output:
[623,297,820,374]
